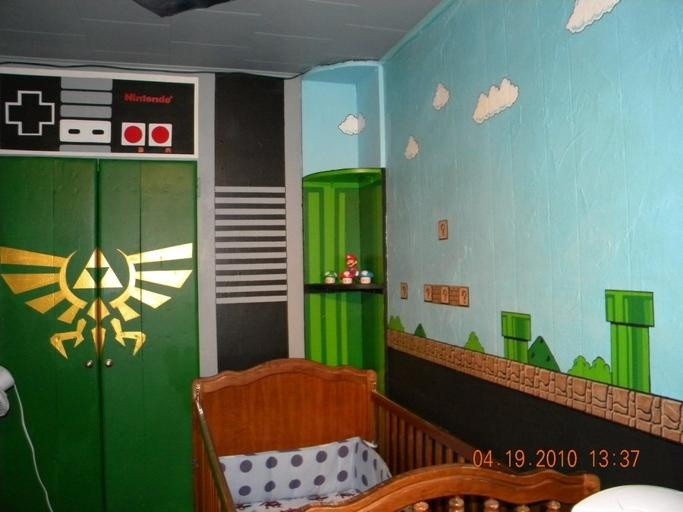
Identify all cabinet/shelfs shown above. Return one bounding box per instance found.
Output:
[0,154,198,512]
[301,167,387,396]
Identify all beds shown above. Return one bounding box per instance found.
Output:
[189,357,601,512]
